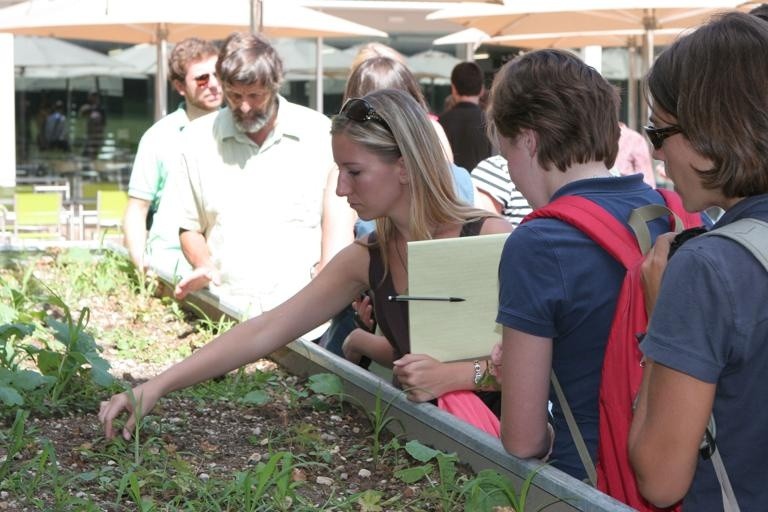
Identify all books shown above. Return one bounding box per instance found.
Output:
[406,232,511,363]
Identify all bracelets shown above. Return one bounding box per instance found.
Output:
[539,422,556,463]
[473,359,483,392]
[310,260,319,279]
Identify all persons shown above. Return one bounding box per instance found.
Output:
[309,56,474,279]
[33,96,106,161]
[123,38,226,296]
[612,120,658,191]
[485,47,711,512]
[97,88,515,443]
[171,31,358,344]
[626,10,768,512]
[437,62,496,175]
[470,151,532,230]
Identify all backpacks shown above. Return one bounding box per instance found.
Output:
[516,188,703,512]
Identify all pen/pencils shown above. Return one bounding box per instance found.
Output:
[385,296,467,302]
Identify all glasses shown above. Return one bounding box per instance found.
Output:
[194,71,217,87]
[340,99,392,132]
[644,124,683,150]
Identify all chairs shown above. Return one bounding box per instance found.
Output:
[78,182,122,241]
[0,182,73,240]
[97,190,127,239]
[13,193,63,240]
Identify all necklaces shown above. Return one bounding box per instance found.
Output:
[395,222,439,278]
